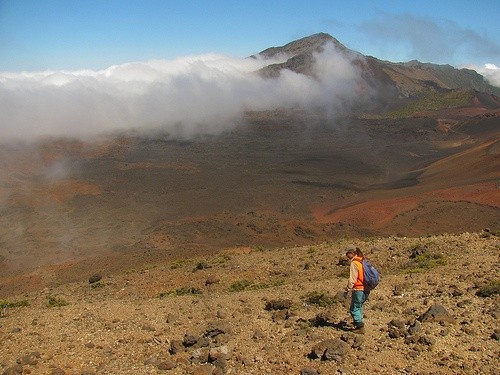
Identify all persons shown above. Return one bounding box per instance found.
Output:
[342,245,370,333]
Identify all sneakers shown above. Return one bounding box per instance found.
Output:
[351,322,364,330]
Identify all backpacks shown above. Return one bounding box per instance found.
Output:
[353,258,379,294]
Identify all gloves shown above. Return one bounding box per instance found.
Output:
[343,288,349,299]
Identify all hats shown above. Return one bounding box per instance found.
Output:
[340,244,356,254]
